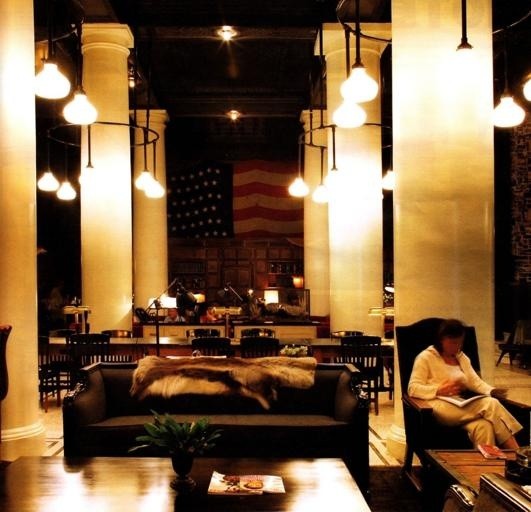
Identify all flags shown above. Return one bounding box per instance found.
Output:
[165,161,302,242]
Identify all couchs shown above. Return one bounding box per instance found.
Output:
[65,361,369,498]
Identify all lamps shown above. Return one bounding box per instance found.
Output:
[34,1,166,198]
[332,0,379,130]
[286,135,311,198]
[494,30,527,131]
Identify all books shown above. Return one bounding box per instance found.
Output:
[436,389,486,407]
[237,473,284,494]
[477,442,506,460]
[207,469,263,495]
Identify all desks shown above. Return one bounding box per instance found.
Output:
[419,444,531,510]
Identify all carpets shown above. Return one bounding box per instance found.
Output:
[357,464,422,511]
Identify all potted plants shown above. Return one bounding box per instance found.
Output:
[125,410,226,490]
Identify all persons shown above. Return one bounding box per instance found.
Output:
[407,318,523,451]
[163,308,186,323]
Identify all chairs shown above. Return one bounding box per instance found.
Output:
[395,315,531,476]
